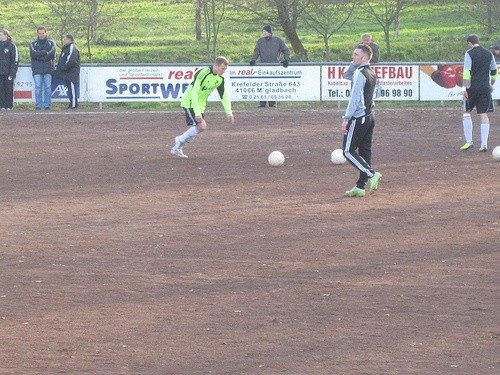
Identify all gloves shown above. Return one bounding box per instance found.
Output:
[250,60,255,66]
[280,60,289,67]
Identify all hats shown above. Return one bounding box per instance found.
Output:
[263,25,271,33]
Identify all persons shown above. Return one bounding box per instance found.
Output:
[55,35,80,110]
[0,29,18,111]
[341,43,382,198]
[170,57,235,159]
[459,35,500,152]
[356,34,380,63]
[29,27,56,110]
[250,25,290,107]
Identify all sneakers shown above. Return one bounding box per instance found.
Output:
[344,186,365,197]
[367,172,382,195]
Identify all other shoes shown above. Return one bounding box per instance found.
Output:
[174,136,184,154]
[36,107,51,111]
[0,107,13,111]
[170,148,188,159]
[478,145,487,152]
[460,142,474,151]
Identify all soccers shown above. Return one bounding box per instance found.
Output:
[492,146,500,161]
[268,151,285,166]
[331,149,347,165]
